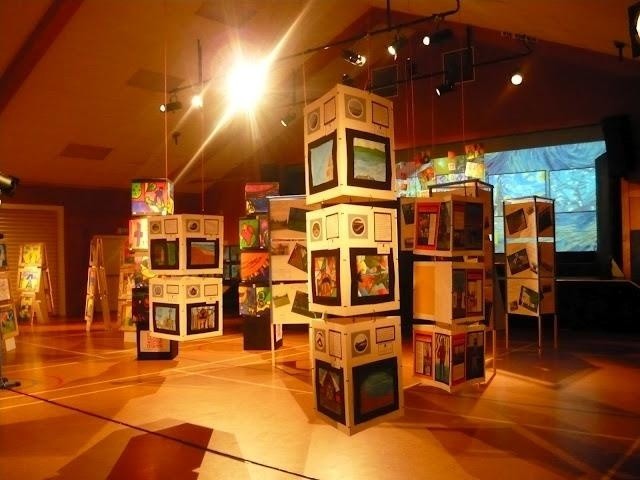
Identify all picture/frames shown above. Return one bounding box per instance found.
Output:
[0,241,44,339]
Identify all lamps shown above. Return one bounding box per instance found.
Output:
[335,12,464,98]
[279,105,298,129]
[159,92,182,113]
[0,176,19,196]
